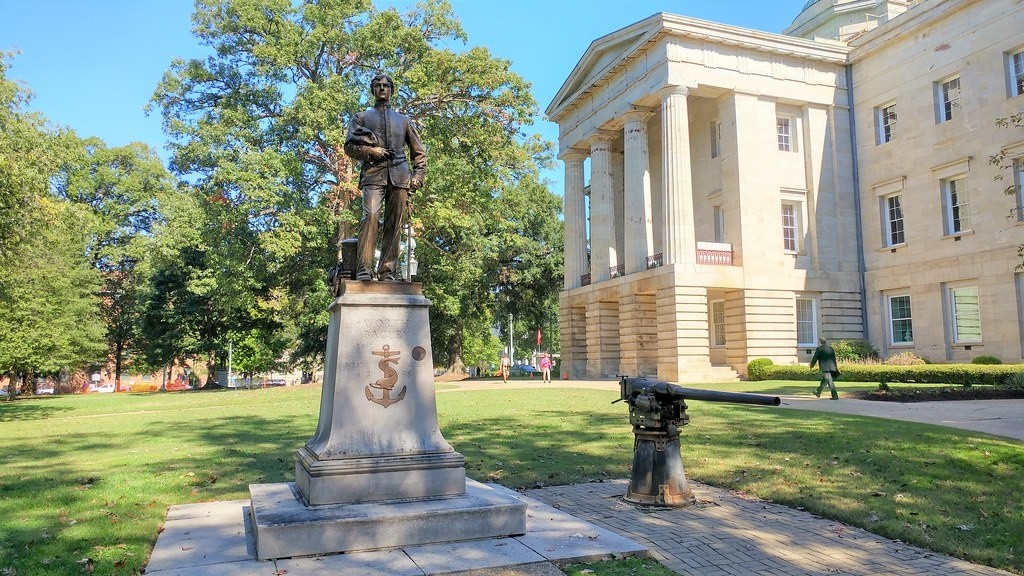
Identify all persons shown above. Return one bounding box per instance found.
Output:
[541,353,551,383]
[477,366,481,376]
[500,353,510,383]
[343,74,428,281]
[83,381,87,388]
[809,336,841,400]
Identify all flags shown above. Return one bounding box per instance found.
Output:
[537,329,542,346]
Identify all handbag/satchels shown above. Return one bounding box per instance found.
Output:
[831,370,840,378]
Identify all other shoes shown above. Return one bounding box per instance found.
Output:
[813,391,820,397]
[830,398,838,400]
